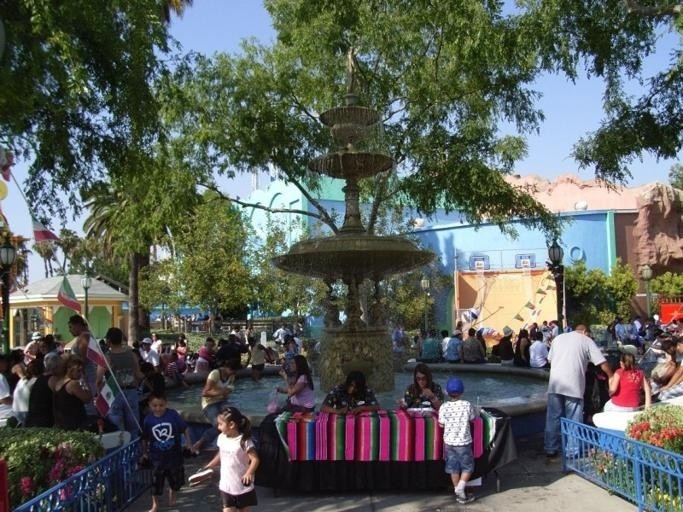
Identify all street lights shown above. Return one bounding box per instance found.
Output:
[420,273,429,334]
[545,237,564,334]
[0,232,16,355]
[80,268,91,321]
[640,264,652,318]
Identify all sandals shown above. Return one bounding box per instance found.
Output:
[188,468,214,488]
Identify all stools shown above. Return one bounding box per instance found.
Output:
[592,411,645,436]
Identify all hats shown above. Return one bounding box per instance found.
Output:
[447,376,464,394]
[503,328,514,337]
[141,338,152,345]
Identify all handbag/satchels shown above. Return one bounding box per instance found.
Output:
[492,345,499,356]
[268,400,282,414]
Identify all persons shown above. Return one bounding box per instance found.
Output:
[275,355,315,412]
[401,364,443,410]
[438,378,478,504]
[193,322,302,371]
[499,314,681,458]
[193,360,242,449]
[204,407,259,512]
[320,371,379,414]
[141,393,191,512]
[1,314,187,458]
[393,321,487,364]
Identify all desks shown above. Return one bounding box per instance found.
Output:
[255,406,517,498]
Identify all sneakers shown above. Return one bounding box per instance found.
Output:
[456,493,475,504]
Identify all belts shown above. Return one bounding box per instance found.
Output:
[121,386,135,390]
